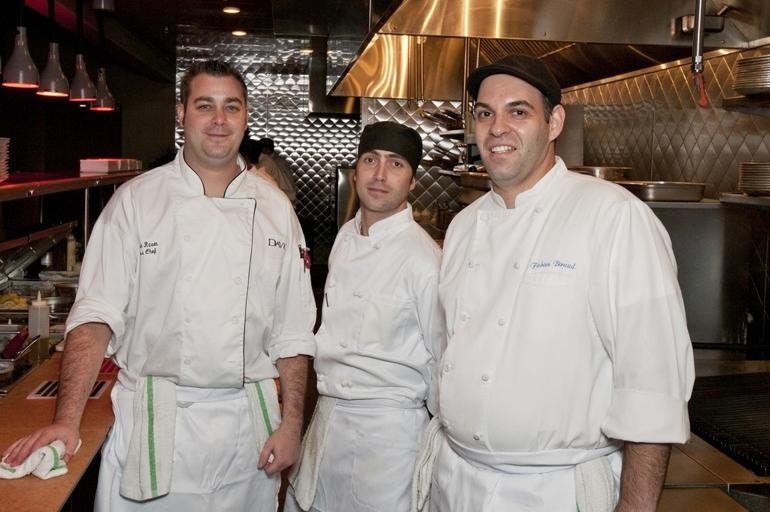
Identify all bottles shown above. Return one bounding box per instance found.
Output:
[27,290,50,364]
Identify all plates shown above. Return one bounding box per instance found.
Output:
[737,160,770,197]
[733,54,770,97]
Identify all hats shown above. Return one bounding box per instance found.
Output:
[465,52,562,105]
[356,122,423,175]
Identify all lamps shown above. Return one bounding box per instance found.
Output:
[1,2,119,114]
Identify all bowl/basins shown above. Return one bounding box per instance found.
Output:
[27,283,79,315]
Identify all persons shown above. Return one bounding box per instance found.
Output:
[0,61,316,511]
[282,121,448,512]
[243,133,296,204]
[409,54,696,512]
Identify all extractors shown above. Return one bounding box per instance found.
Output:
[328,0,770,102]
